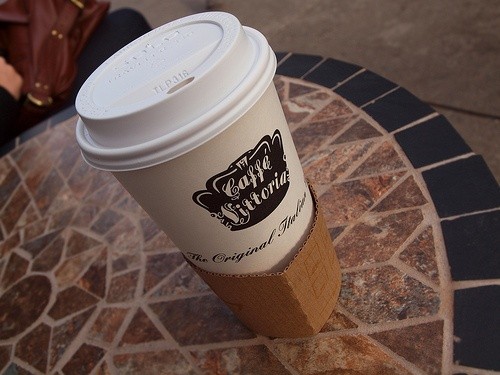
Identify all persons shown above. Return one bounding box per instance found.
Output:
[0,0,150,146]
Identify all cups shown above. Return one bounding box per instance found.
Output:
[75,10,312,275]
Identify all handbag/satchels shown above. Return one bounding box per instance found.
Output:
[0,0,110,120]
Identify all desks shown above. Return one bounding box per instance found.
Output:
[0,51,500,375]
